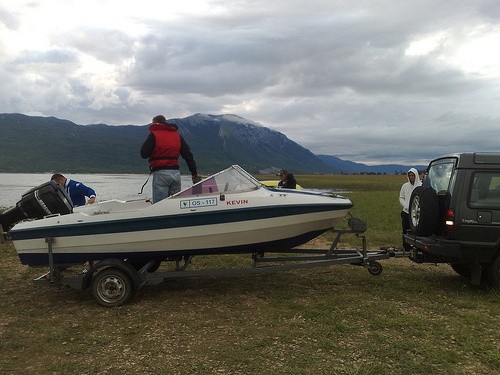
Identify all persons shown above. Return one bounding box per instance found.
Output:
[51,174,96,207]
[276,169,296,189]
[399,168,423,252]
[139,115,198,205]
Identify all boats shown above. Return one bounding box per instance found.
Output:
[0,164,354,267]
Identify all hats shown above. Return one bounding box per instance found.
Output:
[276,169,287,176]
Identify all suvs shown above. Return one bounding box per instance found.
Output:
[402,151,500,289]
